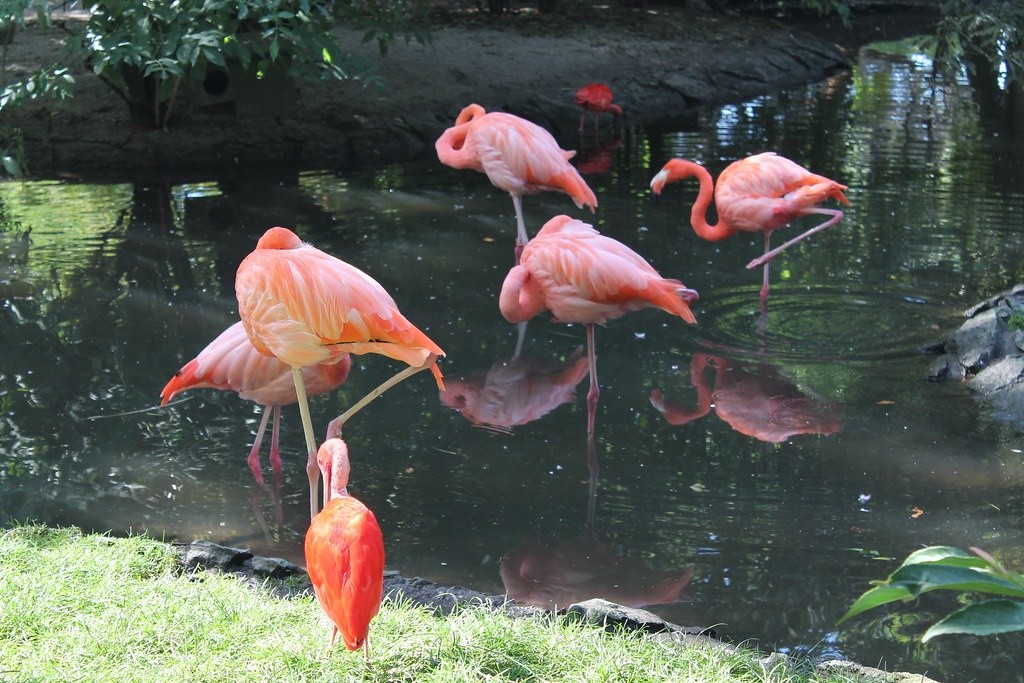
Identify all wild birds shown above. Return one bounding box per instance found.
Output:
[500,214,701,403]
[159,225,446,666]
[652,150,852,303]
[434,105,597,246]
